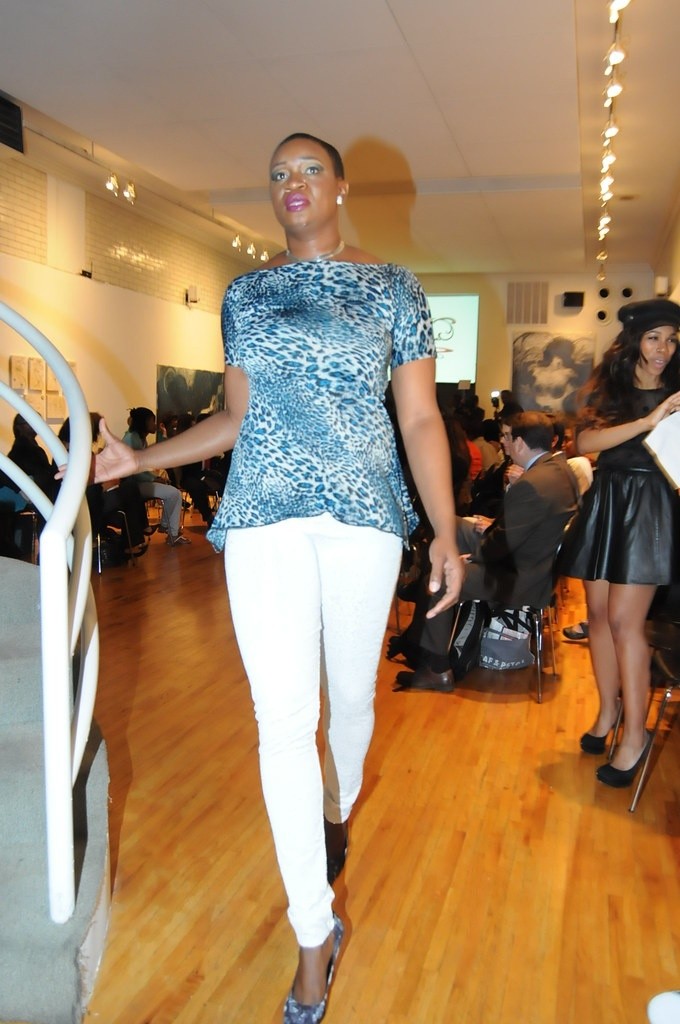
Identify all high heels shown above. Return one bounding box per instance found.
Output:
[280,912,344,1024]
[321,814,351,883]
[136,524,159,538]
[113,543,149,564]
[581,704,625,753]
[597,730,653,787]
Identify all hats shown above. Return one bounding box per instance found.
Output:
[619,299,680,336]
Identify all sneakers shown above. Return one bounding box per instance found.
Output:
[166,534,193,546]
[158,525,184,536]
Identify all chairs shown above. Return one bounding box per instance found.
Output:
[606,620,680,812]
[450,536,576,704]
[18,476,39,565]
[97,510,137,574]
[144,497,175,547]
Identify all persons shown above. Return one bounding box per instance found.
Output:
[553,299,680,790]
[0,389,680,695]
[55,133,466,1024]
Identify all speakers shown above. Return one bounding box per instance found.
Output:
[653,276,668,297]
[188,285,200,303]
[564,292,583,308]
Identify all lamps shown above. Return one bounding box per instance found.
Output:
[231,234,242,253]
[122,183,136,205]
[246,241,256,260]
[594,0,631,281]
[261,247,269,262]
[185,285,198,306]
[104,171,119,197]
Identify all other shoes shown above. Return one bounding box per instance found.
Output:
[389,632,419,648]
[562,622,590,640]
[390,647,426,664]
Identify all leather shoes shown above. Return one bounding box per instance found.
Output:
[395,664,454,692]
[396,577,422,603]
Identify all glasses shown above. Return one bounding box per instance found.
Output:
[499,430,511,442]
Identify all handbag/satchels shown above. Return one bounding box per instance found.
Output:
[479,609,536,670]
